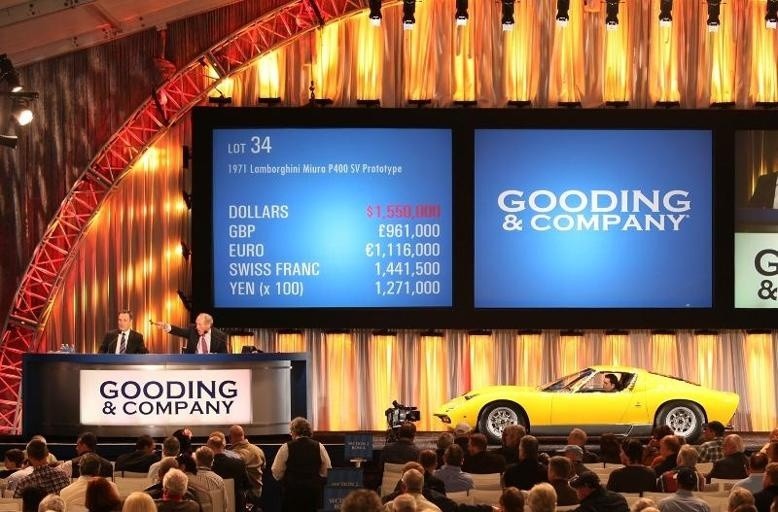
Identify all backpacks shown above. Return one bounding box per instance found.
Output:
[599,433,620,463]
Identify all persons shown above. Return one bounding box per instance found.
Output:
[152,312,228,354]
[602,374,621,392]
[97,309,150,355]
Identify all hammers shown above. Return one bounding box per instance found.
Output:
[149,319,165,329]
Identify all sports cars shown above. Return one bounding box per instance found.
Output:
[433,364,742,445]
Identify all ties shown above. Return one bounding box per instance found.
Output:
[201,334,208,353]
[120,334,126,354]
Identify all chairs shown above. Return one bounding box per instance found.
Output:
[1,462,235,512]
[381,462,745,512]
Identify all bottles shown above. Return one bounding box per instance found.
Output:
[59,343,75,354]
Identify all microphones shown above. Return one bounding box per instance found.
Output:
[204,330,232,354]
[123,333,131,354]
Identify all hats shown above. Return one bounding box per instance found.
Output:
[455,423,472,434]
[673,468,697,485]
[568,471,599,488]
[556,445,583,454]
